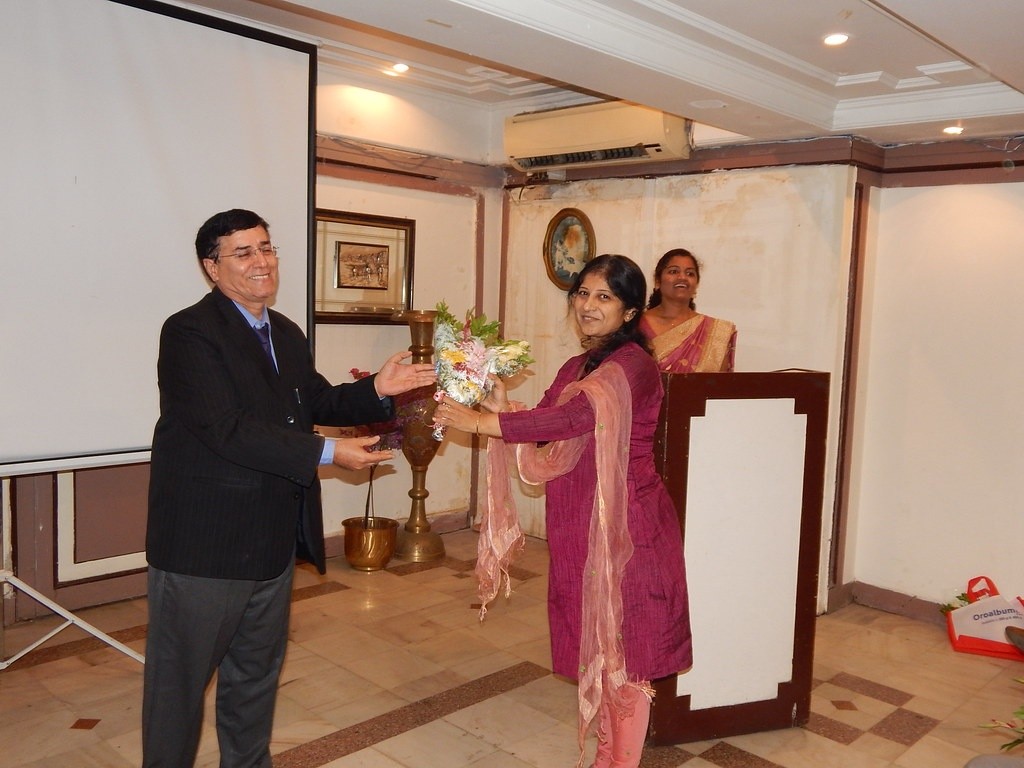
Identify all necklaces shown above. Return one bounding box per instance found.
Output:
[668,309,692,335]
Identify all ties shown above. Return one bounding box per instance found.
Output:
[252,322,272,358]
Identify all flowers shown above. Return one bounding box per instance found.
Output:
[433,297,536,441]
[340,367,425,529]
[936,591,970,616]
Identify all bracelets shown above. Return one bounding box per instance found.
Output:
[476,413,482,438]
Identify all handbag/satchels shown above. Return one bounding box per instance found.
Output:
[945,575,1024,662]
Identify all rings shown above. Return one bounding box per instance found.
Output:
[447,405,452,411]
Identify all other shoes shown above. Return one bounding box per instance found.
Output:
[1004,625,1024,651]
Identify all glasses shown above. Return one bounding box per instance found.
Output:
[209,244,280,261]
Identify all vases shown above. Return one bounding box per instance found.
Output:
[341,516,400,572]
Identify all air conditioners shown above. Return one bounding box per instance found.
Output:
[502,98,690,173]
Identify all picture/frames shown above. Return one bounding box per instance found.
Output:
[543,208,596,291]
[315,207,416,326]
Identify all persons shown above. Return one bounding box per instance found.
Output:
[638,248,735,373]
[430,254,693,768]
[140,209,437,768]
